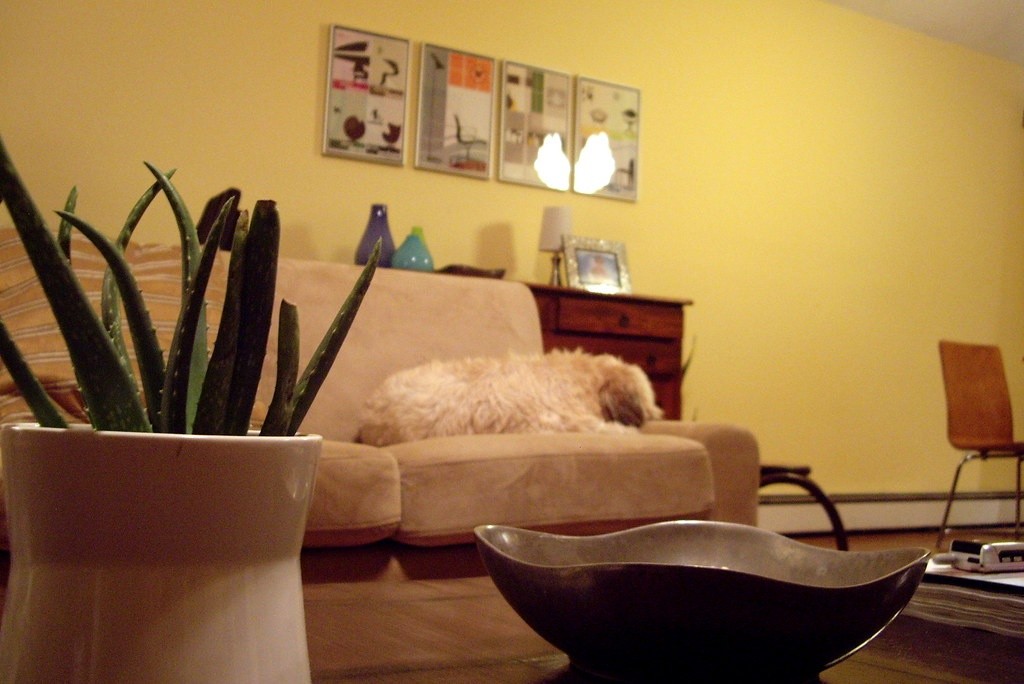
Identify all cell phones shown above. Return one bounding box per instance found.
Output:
[933,539,1024,573]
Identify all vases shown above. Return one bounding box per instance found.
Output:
[391,227,432,272]
[355,203,394,267]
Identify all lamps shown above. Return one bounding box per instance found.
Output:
[538,207,572,286]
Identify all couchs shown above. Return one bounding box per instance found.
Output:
[0,227,760,583]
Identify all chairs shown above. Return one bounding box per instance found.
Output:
[936,342,1024,550]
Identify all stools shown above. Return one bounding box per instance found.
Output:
[759,463,847,553]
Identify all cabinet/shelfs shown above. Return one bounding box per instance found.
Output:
[524,282,693,420]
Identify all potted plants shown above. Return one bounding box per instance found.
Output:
[0,137,383,684]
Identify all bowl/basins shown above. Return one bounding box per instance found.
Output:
[467,514,933,674]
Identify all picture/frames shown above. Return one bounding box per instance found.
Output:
[570,75,641,201]
[563,234,633,294]
[415,41,497,180]
[322,24,411,167]
[499,61,574,191]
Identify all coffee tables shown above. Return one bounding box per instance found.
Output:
[304,575,1024,684]
